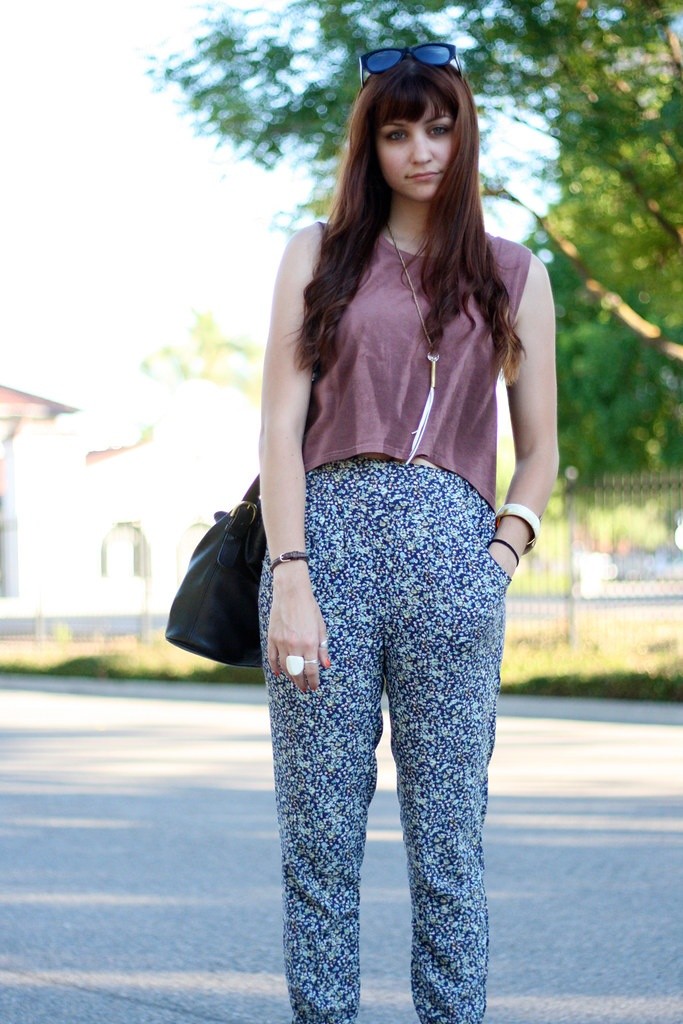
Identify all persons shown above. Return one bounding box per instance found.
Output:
[254,45,562,1024]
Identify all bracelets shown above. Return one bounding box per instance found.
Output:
[268,551,310,573]
[494,503,541,557]
[486,539,520,567]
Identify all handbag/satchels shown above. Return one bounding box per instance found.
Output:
[166,473,266,671]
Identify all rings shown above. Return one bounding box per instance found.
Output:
[285,655,304,676]
[320,641,329,649]
[303,660,320,664]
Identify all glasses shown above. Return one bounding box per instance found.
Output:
[359,41,462,90]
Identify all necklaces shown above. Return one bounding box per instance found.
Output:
[386,220,443,468]
